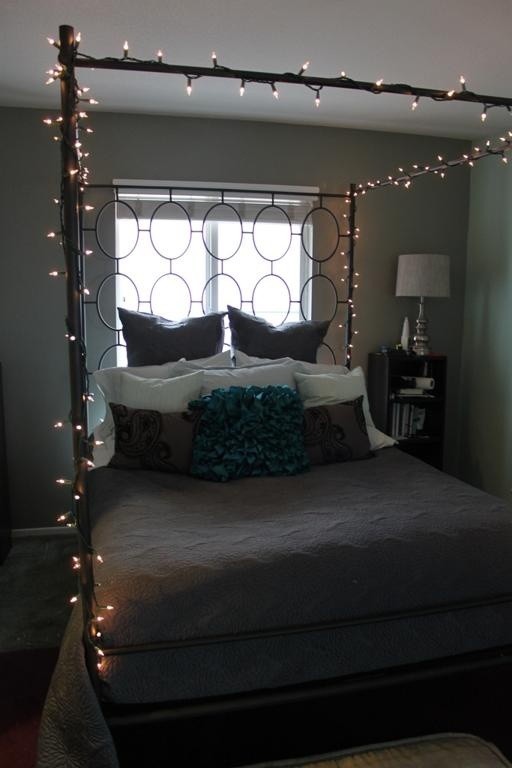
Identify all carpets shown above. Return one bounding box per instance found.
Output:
[2,535,76,651]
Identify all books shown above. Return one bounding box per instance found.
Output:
[397,388,425,395]
[389,402,430,441]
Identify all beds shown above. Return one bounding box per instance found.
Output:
[62,185,508,765]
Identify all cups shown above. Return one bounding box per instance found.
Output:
[380,345,390,353]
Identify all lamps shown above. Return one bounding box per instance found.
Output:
[395,253,451,355]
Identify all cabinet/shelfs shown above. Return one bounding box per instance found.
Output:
[370,352,446,471]
